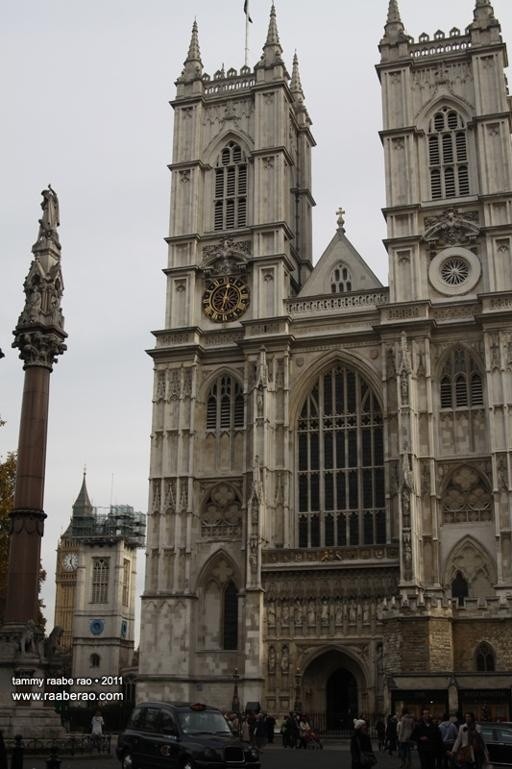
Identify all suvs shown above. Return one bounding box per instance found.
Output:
[114,700,261,769]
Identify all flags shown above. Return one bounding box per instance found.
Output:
[244,0,253,24]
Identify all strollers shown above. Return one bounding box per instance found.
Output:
[302,729,323,754]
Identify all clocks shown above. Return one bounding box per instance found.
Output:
[62,551,79,572]
[202,277,251,323]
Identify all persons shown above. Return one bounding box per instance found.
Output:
[91,710,105,736]
[29,285,41,322]
[373,705,490,769]
[222,707,324,751]
[50,290,60,327]
[350,717,378,769]
[36,183,60,243]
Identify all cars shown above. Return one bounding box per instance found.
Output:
[475,721,512,769]
